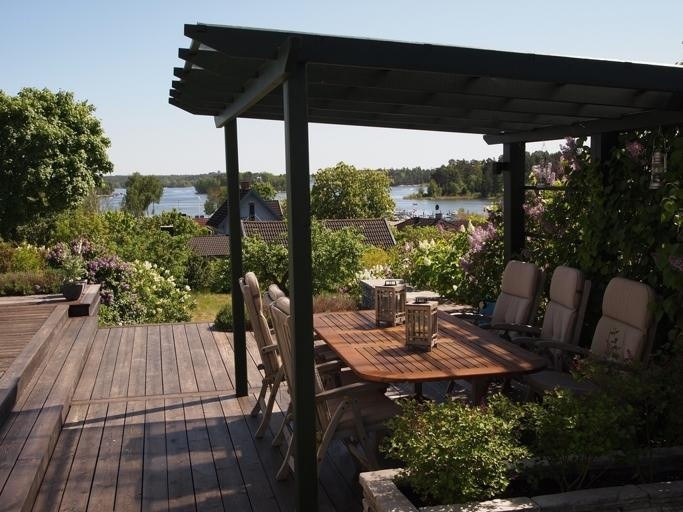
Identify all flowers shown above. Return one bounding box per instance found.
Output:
[45,238,104,284]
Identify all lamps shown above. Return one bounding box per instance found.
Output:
[405,298,438,352]
[375,281,406,326]
[649,127,668,190]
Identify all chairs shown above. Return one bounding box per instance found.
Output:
[238,272,403,481]
[446,260,664,402]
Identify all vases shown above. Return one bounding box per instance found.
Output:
[62,279,87,300]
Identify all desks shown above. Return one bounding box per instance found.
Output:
[313,309,548,402]
